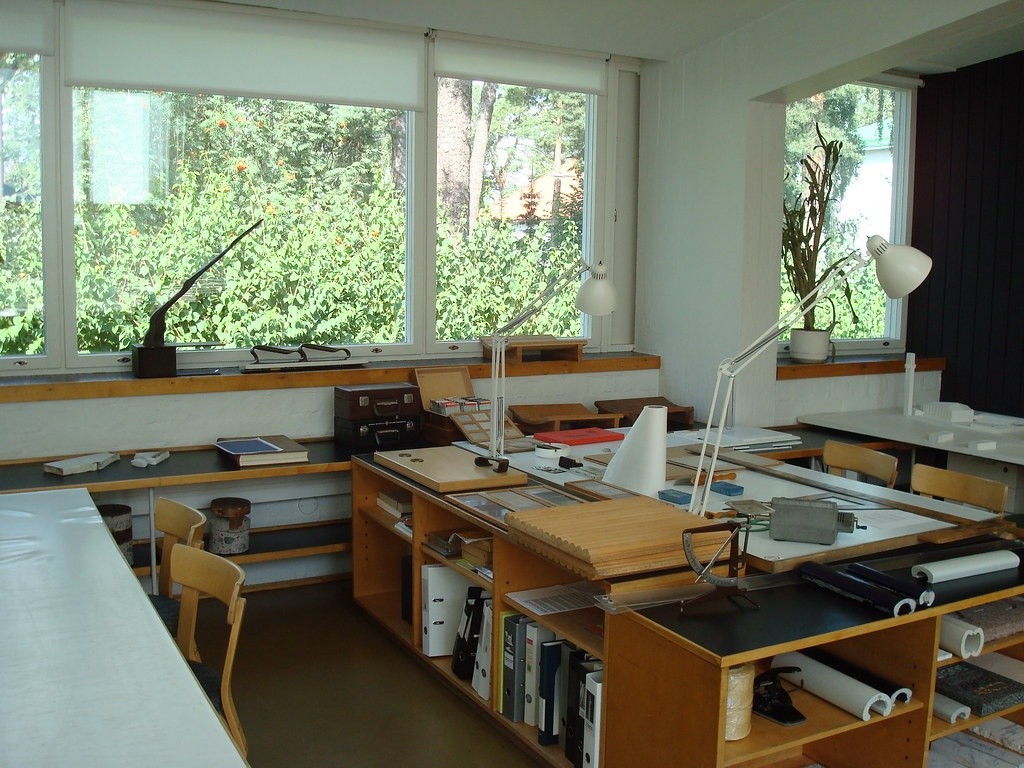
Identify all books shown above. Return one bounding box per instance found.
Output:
[218,435,311,467]
[422,527,493,583]
[376,489,413,536]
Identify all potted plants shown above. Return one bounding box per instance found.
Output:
[781,122,858,363]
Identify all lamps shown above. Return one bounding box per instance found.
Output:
[490,256,616,458]
[686,235,932,517]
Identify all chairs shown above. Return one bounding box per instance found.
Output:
[148,496,209,663]
[171,543,248,758]
[911,463,1009,514]
[823,439,898,489]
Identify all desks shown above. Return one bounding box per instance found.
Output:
[0,487,252,767]
[798,402,1024,468]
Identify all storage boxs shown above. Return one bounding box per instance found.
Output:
[334,381,421,420]
[418,421,467,446]
[413,365,526,446]
[334,417,422,452]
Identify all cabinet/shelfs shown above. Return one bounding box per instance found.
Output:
[0,420,1024,767]
